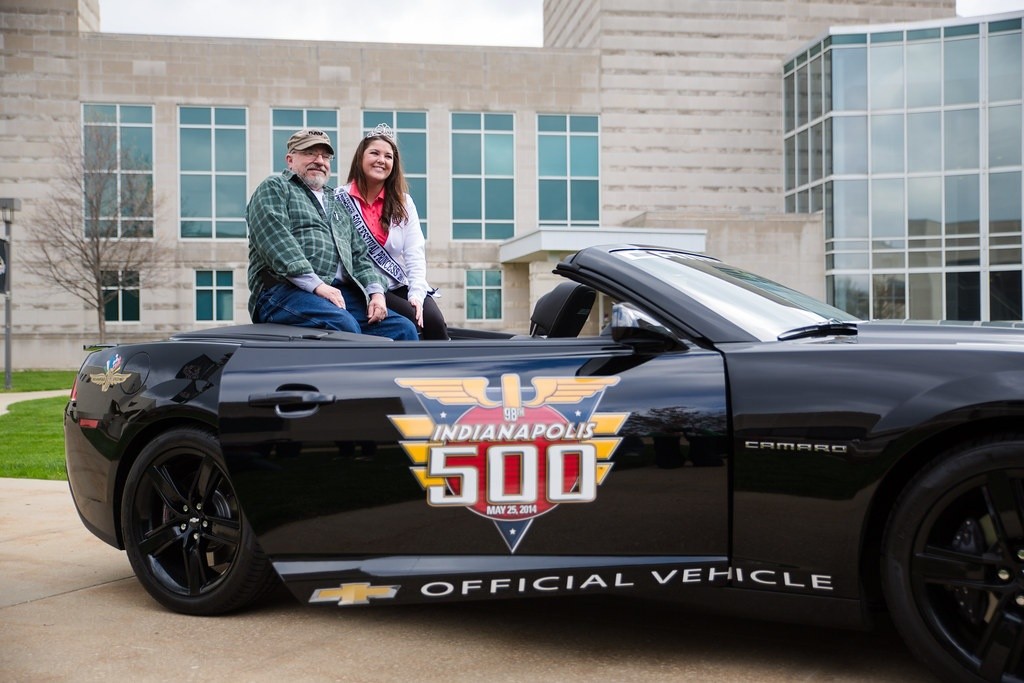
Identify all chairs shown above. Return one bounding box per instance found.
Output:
[510,280,597,340]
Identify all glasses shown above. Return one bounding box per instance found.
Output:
[291,150,335,162]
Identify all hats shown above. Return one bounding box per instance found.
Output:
[286,130,335,156]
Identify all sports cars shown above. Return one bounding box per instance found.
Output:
[63,241,1024,682]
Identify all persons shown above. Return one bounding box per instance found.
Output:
[244,129,420,343]
[335,123,451,342]
